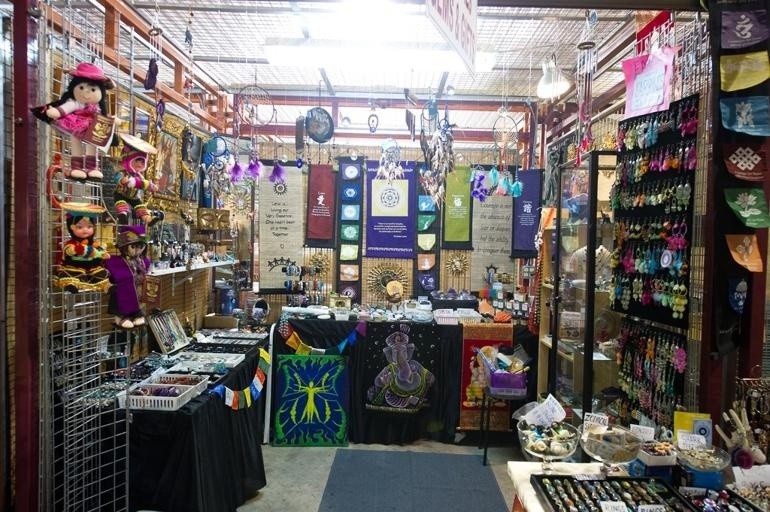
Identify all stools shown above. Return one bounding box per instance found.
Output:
[479,387,530,467]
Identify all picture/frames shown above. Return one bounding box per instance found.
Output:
[109,93,203,204]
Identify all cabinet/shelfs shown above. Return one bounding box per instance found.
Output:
[537,147,620,463]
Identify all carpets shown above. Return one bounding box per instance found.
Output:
[315,447,508,512]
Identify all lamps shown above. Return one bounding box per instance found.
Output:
[536,52,572,100]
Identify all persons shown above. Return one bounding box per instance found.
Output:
[29,63,159,330]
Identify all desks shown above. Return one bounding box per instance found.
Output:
[83,324,273,512]
[508,460,770,512]
[273,305,536,444]
